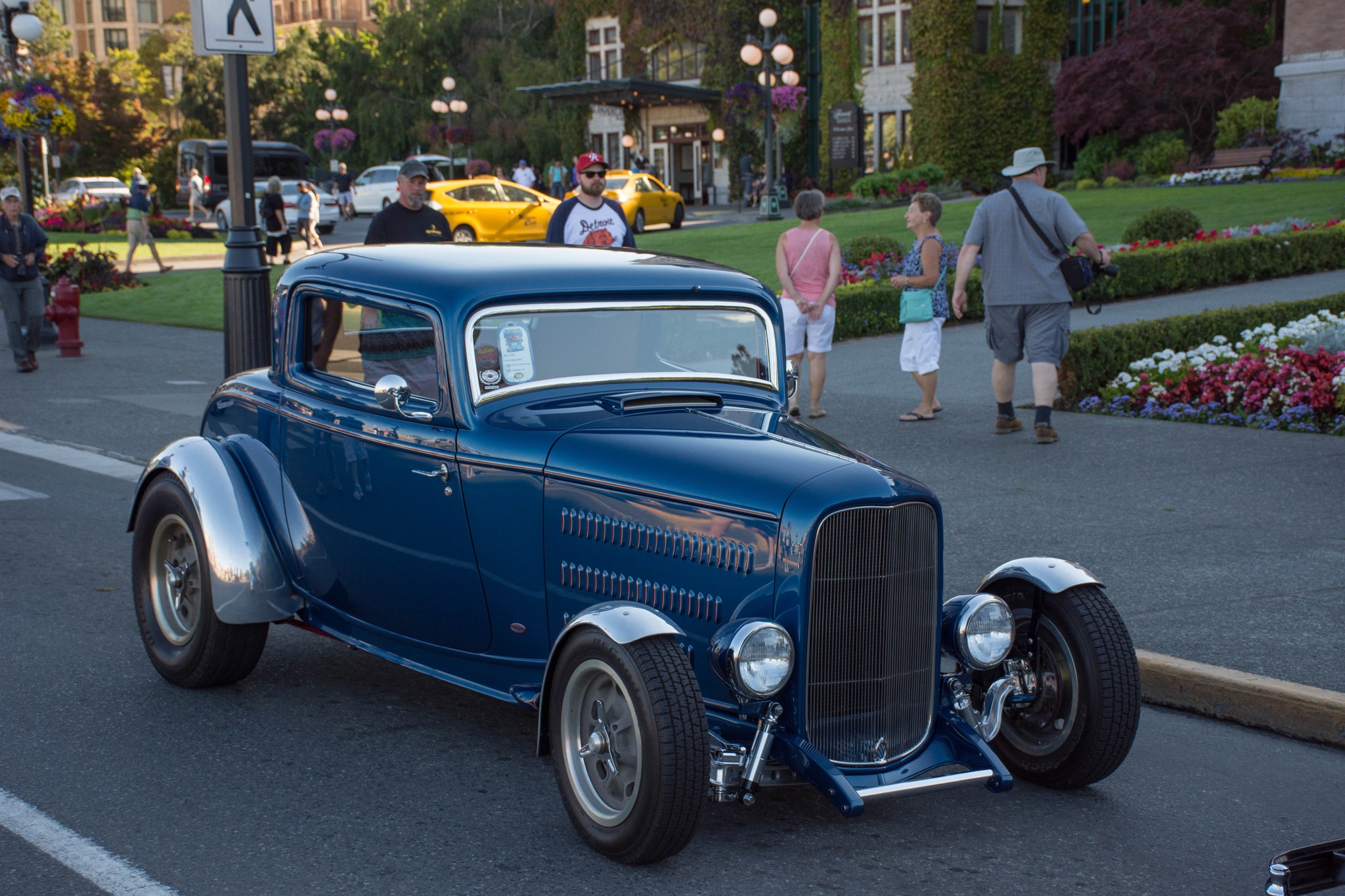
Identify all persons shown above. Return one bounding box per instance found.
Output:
[0,187,48,371]
[775,189,839,418]
[546,152,637,318]
[360,160,455,328]
[952,147,1110,442]
[126,166,173,274]
[259,176,292,265]
[293,154,784,250]
[186,168,211,221]
[891,191,952,420]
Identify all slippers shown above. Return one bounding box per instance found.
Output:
[932,407,942,413]
[899,411,934,421]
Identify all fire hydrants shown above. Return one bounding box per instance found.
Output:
[44,276,84,357]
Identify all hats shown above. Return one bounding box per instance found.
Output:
[576,153,610,173]
[1002,146,1057,177]
[398,158,429,180]
[0,186,21,200]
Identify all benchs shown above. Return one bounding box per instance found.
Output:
[1197,144,1279,183]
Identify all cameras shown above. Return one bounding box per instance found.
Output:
[1091,261,1119,276]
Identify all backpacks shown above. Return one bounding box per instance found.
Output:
[259,191,276,224]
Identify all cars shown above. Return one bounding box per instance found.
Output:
[214,180,337,236]
[126,244,1144,865]
[54,177,134,206]
[340,153,686,247]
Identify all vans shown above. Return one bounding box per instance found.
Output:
[175,131,310,208]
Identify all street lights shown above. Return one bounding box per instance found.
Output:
[0,1,43,217]
[316,90,348,182]
[432,78,467,180]
[738,10,799,221]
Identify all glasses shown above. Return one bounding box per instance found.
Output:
[579,170,606,178]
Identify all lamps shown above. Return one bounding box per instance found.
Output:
[620,134,635,148]
[711,126,725,141]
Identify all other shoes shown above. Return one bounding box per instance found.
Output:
[1034,424,1057,442]
[124,272,137,276]
[27,351,38,369]
[185,217,195,222]
[810,409,826,418]
[205,213,212,221]
[161,266,174,273]
[17,361,33,372]
[995,414,1022,434]
[789,407,800,416]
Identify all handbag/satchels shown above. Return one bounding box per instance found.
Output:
[899,289,935,324]
[1059,256,1094,292]
